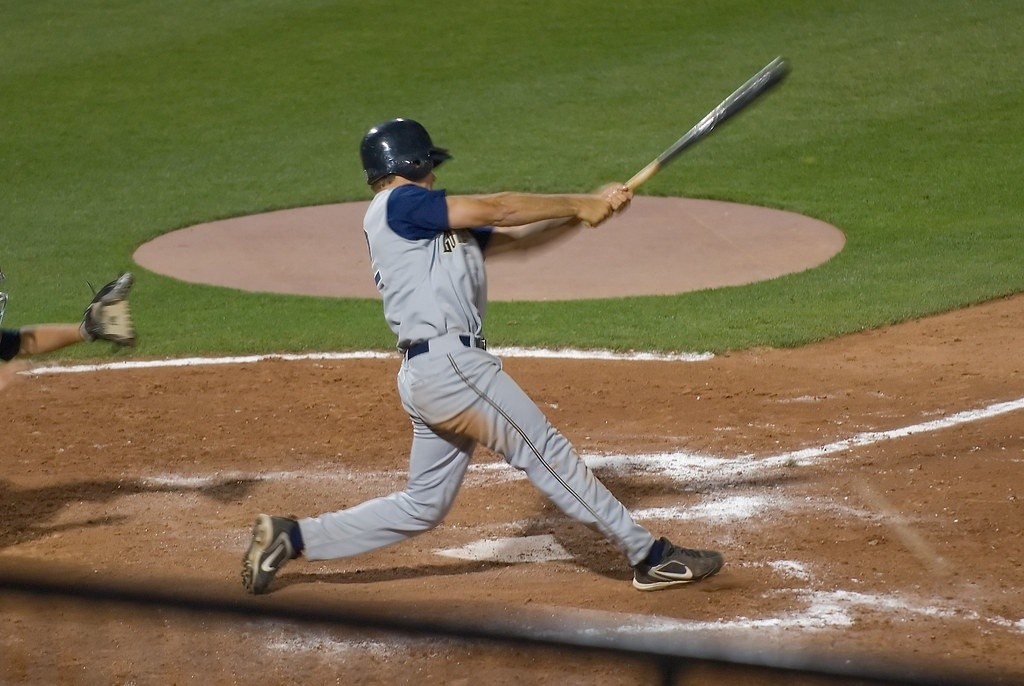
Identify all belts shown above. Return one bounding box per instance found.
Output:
[407,336,487,360]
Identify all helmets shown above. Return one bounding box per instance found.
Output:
[360,117,453,184]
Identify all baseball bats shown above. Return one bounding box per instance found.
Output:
[582,53,793,229]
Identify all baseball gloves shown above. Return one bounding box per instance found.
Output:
[78,269,140,350]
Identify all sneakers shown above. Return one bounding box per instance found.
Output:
[632,537,723,590]
[241,514,300,594]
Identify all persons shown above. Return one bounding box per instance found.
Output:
[0,268,136,363]
[241,119,723,594]
